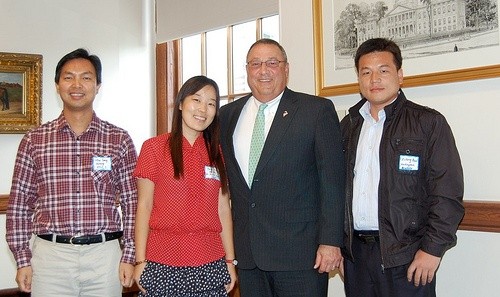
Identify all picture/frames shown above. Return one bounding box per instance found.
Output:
[0,52,43,135]
[312,0,500,98]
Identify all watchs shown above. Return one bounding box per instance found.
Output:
[225,259,239,266]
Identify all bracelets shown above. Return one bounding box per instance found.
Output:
[134,259,149,266]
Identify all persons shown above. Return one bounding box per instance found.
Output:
[339,38,465,297]
[218,39,348,297]
[6,49,138,297]
[134,76,238,297]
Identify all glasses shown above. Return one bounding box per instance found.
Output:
[247,59,286,69]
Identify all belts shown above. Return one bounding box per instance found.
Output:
[354,234,380,245]
[35,232,125,245]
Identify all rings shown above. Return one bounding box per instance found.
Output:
[335,265,340,269]
[332,265,336,269]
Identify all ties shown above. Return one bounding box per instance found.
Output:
[248,104,268,189]
